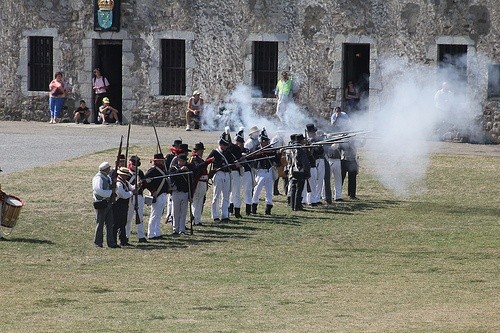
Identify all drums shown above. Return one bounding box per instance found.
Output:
[1,195,23,229]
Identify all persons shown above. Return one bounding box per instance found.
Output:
[92,124,369,248]
[274,70,298,122]
[345,80,360,111]
[97,97,119,125]
[48,72,65,124]
[185,91,206,131]
[434,82,454,111]
[330,107,350,126]
[73,100,91,124]
[92,67,110,124]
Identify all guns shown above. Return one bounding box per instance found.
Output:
[142,171,192,184]
[151,123,162,154]
[133,165,142,226]
[216,129,378,173]
[111,135,124,205]
[125,123,132,168]
[186,174,195,235]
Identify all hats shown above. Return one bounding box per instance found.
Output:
[103,97,109,103]
[175,154,188,161]
[115,154,125,162]
[249,126,260,136]
[193,91,201,95]
[179,144,191,152]
[290,133,297,143]
[193,143,206,150]
[117,166,131,177]
[99,161,111,170]
[219,126,231,146]
[259,127,270,141]
[129,156,141,167]
[172,140,182,147]
[154,153,165,161]
[295,134,305,143]
[305,124,317,132]
[236,128,245,143]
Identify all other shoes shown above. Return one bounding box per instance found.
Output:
[94,216,230,248]
[102,121,109,125]
[116,121,122,125]
[48,119,56,123]
[273,192,358,211]
[75,120,79,124]
[185,126,191,131]
[83,120,90,124]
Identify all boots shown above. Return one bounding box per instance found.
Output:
[229,201,273,219]
